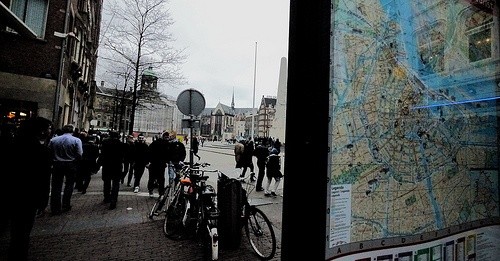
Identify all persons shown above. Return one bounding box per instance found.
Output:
[47,122,187,217]
[191,137,201,161]
[232,135,283,196]
[0,116,51,261]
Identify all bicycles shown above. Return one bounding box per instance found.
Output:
[149,149,276,261]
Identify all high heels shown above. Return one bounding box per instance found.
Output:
[250,178,256,182]
[239,179,246,183]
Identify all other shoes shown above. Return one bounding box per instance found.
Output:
[82,189,86,194]
[260,187,264,190]
[199,157,200,160]
[63,206,72,210]
[103,200,109,203]
[134,186,139,192]
[150,195,154,197]
[158,195,163,200]
[272,192,277,197]
[110,203,116,209]
[127,183,130,187]
[264,193,271,196]
[52,210,62,213]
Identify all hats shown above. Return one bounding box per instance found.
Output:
[271,148,278,153]
[128,135,134,138]
